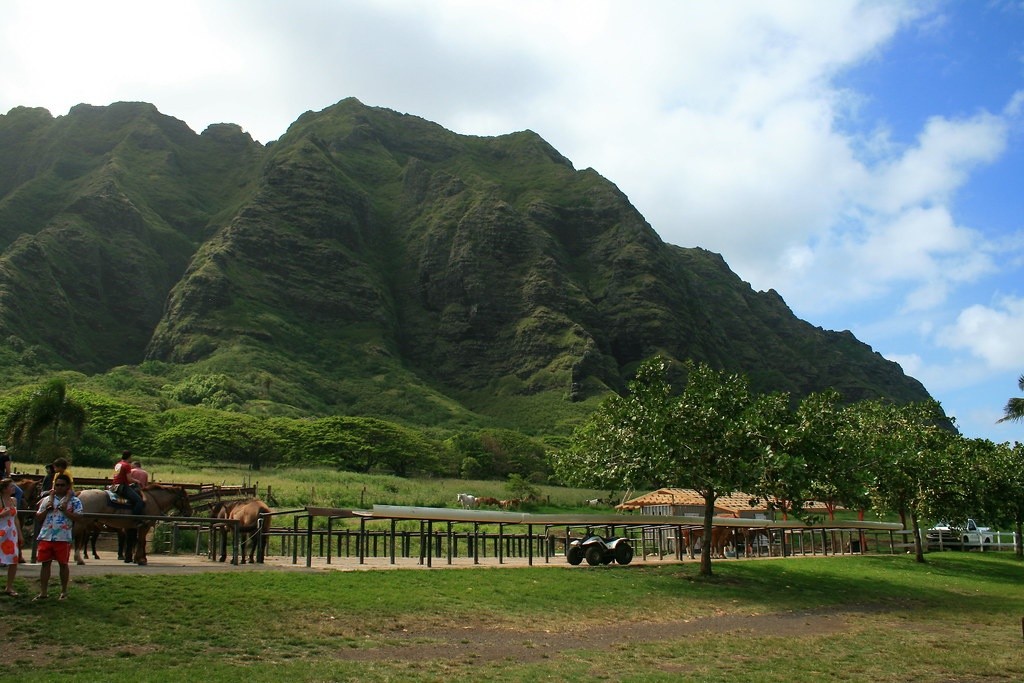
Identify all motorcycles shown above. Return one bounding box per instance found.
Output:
[566,530,633,566]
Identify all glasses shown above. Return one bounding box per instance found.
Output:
[55,483,67,487]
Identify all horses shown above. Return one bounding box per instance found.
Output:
[680,516,776,558]
[76,486,192,563]
[479,497,499,516]
[210,500,270,563]
[585,498,604,507]
[457,493,475,508]
[502,500,523,511]
[14,478,41,560]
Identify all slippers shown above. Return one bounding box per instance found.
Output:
[5,588,18,596]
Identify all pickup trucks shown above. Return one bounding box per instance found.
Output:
[924,518,993,552]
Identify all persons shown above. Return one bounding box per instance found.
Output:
[31,457,83,602]
[0,445,26,596]
[111,451,149,514]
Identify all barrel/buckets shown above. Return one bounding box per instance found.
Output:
[851,540,859,552]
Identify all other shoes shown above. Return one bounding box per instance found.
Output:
[58,593,66,601]
[32,595,49,602]
